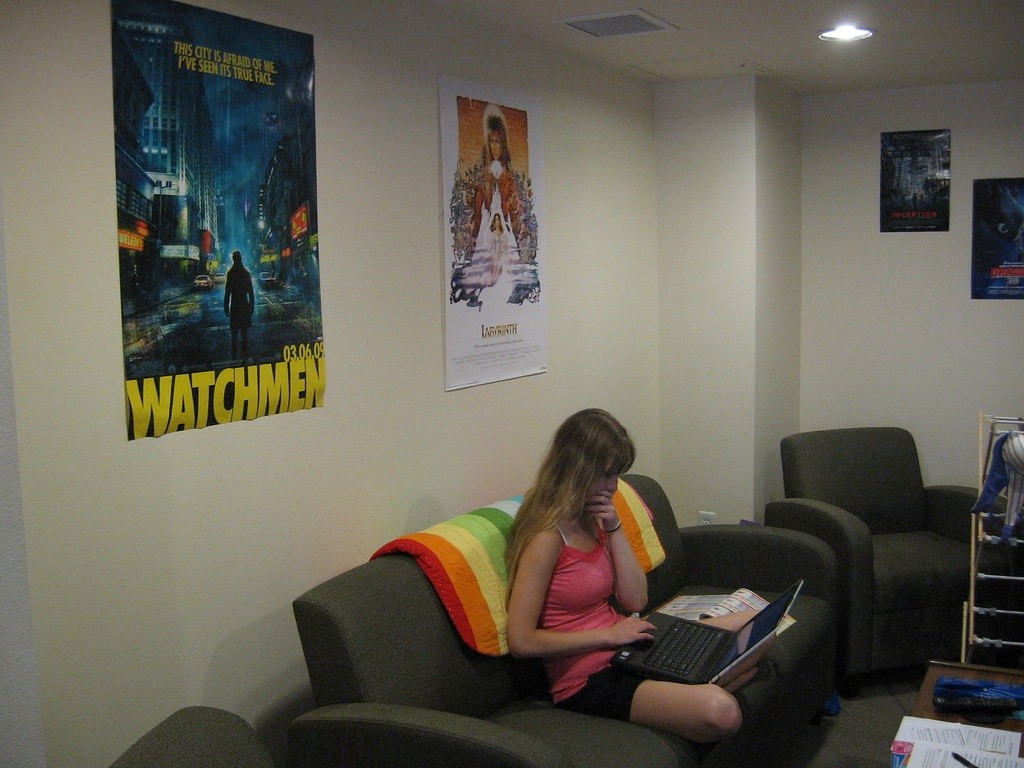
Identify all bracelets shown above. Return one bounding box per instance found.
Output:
[603,520,621,533]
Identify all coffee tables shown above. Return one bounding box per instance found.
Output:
[911,659,1024,768]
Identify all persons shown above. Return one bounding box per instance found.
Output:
[504,407,777,745]
[463,115,526,299]
[222,248,255,362]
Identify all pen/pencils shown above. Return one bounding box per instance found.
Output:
[951,752,979,768]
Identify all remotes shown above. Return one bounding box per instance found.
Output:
[934,697,1024,713]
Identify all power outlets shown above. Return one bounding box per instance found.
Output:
[698,511,716,525]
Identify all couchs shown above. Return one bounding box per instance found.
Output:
[764,424,980,700]
[109,704,276,768]
[284,474,840,768]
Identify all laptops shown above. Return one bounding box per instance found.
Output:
[610,579,803,685]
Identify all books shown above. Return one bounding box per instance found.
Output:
[645,587,798,640]
